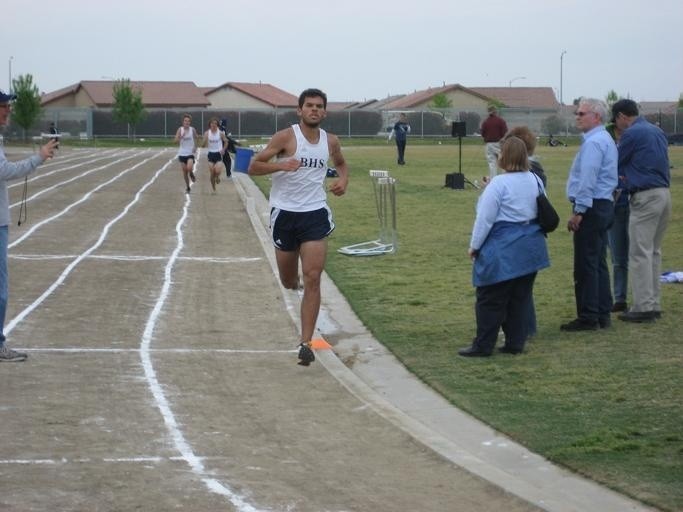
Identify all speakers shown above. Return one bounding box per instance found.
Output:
[452,122,466,137]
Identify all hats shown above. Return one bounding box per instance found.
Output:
[0,92,21,104]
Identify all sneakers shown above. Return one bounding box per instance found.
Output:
[183,171,232,196]
[295,344,316,366]
[1,348,30,364]
[610,300,629,312]
[558,312,612,332]
[456,341,493,357]
[497,346,523,355]
[616,308,658,323]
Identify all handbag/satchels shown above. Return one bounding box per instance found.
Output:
[532,172,561,234]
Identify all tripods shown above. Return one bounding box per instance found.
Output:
[441,138,479,190]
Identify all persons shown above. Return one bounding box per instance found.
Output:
[50,123,58,149]
[389,112,411,165]
[0,91,60,362]
[175,114,242,194]
[560,98,672,331]
[248,88,349,366]
[458,137,550,356]
[480,105,508,178]
[480,126,546,342]
[547,134,568,147]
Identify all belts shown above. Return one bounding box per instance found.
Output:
[628,186,650,196]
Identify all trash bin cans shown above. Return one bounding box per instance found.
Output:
[234,148,254,174]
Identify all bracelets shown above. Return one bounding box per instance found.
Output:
[575,210,584,216]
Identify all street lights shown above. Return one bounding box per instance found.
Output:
[7,54,15,95]
[559,49,568,105]
[508,75,526,87]
